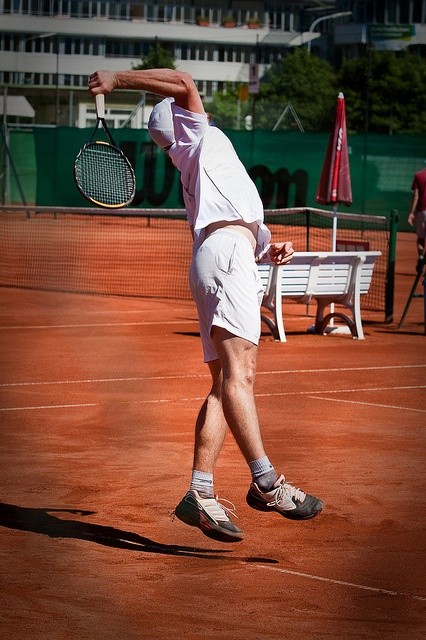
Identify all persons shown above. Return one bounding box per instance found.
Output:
[408,167,426,272]
[87,69,325,541]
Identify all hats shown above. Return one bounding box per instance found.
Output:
[148,96,176,148]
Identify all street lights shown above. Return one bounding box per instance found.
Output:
[306,12,351,54]
[20,33,56,84]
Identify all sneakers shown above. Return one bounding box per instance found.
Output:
[174,489,245,542]
[246,474,323,520]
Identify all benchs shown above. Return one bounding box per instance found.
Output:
[256,250,384,343]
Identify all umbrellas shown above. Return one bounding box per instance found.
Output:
[309,92,356,334]
